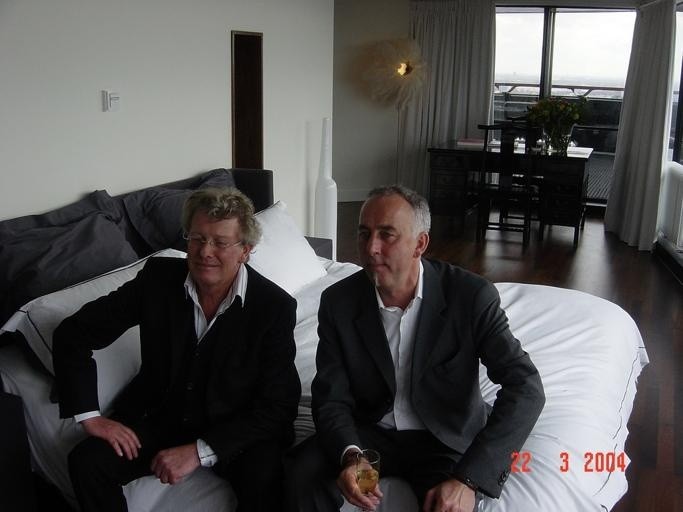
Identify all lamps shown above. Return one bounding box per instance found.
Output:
[363,31,429,110]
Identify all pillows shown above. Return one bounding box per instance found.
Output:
[0,248,187,415]
[124,167,236,253]
[246,200,329,296]
[0,189,140,345]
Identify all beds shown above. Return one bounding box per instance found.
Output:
[0,167,651,511]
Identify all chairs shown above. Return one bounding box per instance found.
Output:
[475,118,546,256]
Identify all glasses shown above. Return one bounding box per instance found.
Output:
[183,233,242,249]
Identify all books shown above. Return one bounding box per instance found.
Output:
[456,138,488,147]
[488,140,518,148]
[566,148,588,155]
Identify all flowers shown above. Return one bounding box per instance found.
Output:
[524,94,589,148]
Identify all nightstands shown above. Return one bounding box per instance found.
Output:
[305,237,333,260]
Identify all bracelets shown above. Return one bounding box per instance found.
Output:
[451,472,479,495]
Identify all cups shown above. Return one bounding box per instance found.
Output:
[356,449,380,494]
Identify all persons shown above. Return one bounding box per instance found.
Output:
[50,186,300,512]
[278,183,545,512]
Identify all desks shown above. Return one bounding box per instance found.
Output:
[426,139,596,247]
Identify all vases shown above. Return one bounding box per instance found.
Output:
[547,133,572,161]
